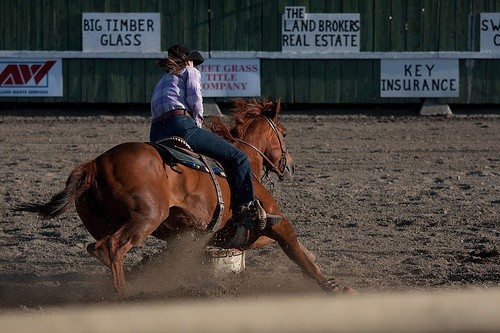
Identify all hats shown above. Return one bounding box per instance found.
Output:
[158,45,204,68]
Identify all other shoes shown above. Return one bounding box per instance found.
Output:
[253,211,282,227]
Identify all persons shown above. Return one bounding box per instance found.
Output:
[149,44,283,230]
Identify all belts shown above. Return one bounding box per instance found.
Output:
[152,110,193,123]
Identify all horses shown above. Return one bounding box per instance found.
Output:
[10,96,358,299]
